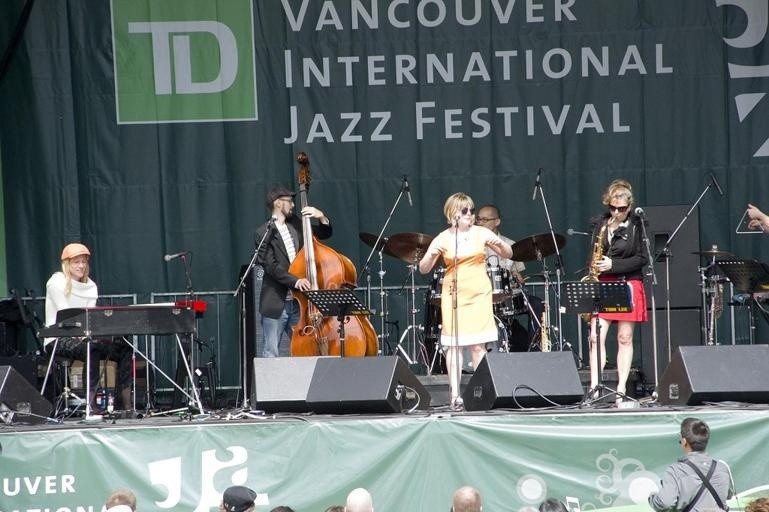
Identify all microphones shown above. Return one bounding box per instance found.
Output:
[567,228,591,237]
[633,207,647,219]
[405,180,413,206]
[710,173,724,197]
[532,174,541,200]
[455,218,459,229]
[164,251,187,262]
[267,214,278,227]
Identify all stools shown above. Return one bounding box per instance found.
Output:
[48,351,108,419]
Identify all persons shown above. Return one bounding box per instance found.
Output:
[647,418,733,512]
[326,487,376,512]
[586,178,649,403]
[452,485,483,512]
[106,489,136,511]
[747,201,769,235]
[475,203,527,282]
[272,506,295,512]
[539,497,565,512]
[418,192,513,409]
[42,242,143,418]
[745,498,769,512]
[254,188,332,356]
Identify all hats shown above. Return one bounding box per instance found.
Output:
[61,243,91,260]
[266,184,297,205]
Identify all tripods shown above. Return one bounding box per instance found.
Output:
[578,303,637,408]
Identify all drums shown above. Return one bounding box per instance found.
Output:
[485,267,511,305]
[434,313,511,375]
[429,269,445,307]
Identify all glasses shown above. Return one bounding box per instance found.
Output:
[609,203,630,212]
[462,207,476,215]
[476,217,495,223]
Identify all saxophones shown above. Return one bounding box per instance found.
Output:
[578,217,617,322]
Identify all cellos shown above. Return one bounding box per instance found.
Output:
[288,153,382,355]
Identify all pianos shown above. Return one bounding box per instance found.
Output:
[40,308,195,338]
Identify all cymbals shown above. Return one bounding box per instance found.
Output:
[511,232,566,261]
[691,251,734,256]
[359,233,400,258]
[387,233,436,264]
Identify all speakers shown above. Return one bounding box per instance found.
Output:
[640,204,702,391]
[306,356,432,414]
[250,355,342,414]
[462,351,584,411]
[0,364,54,427]
[659,343,769,406]
